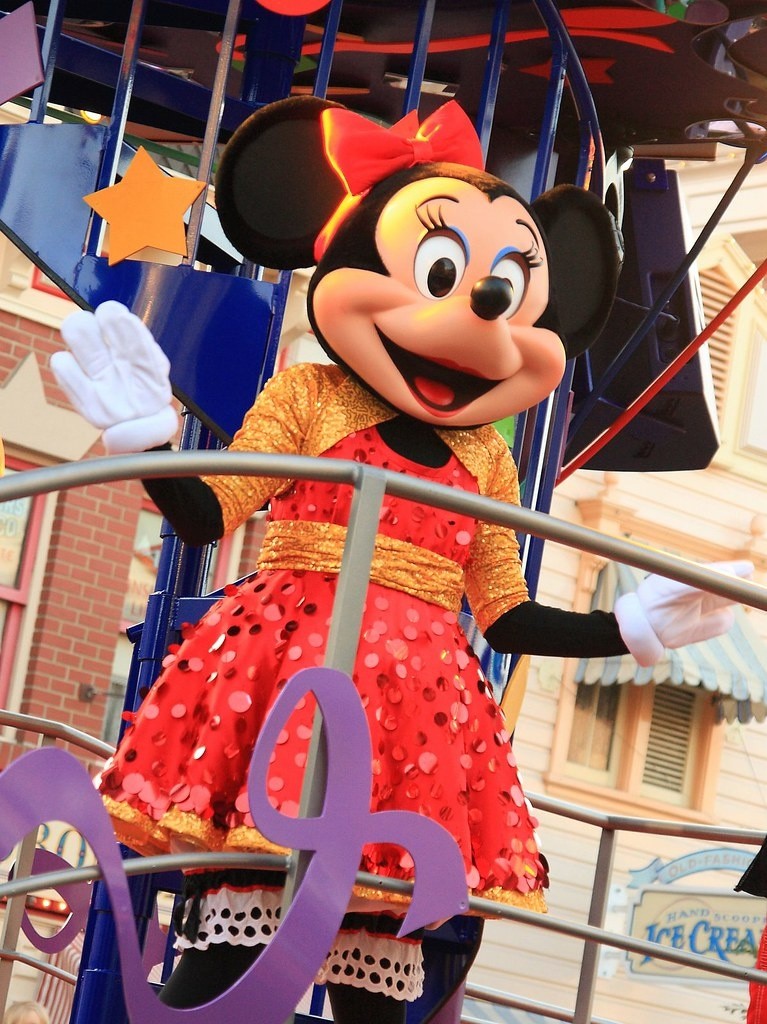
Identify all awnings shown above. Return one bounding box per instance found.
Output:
[573,560,767,725]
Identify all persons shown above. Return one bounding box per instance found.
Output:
[50,93,757,1024]
